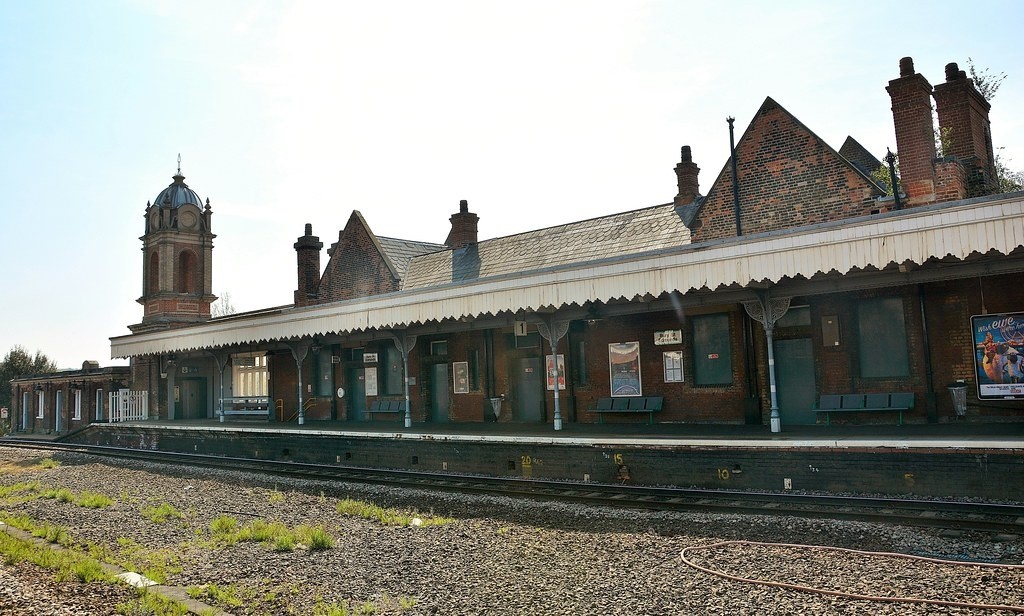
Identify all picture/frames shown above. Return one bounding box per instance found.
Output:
[663,351,685,383]
[608,341,642,398]
[453,362,469,393]
[546,354,566,390]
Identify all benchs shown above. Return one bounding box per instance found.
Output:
[587,397,665,425]
[361,401,411,422]
[811,392,914,426]
